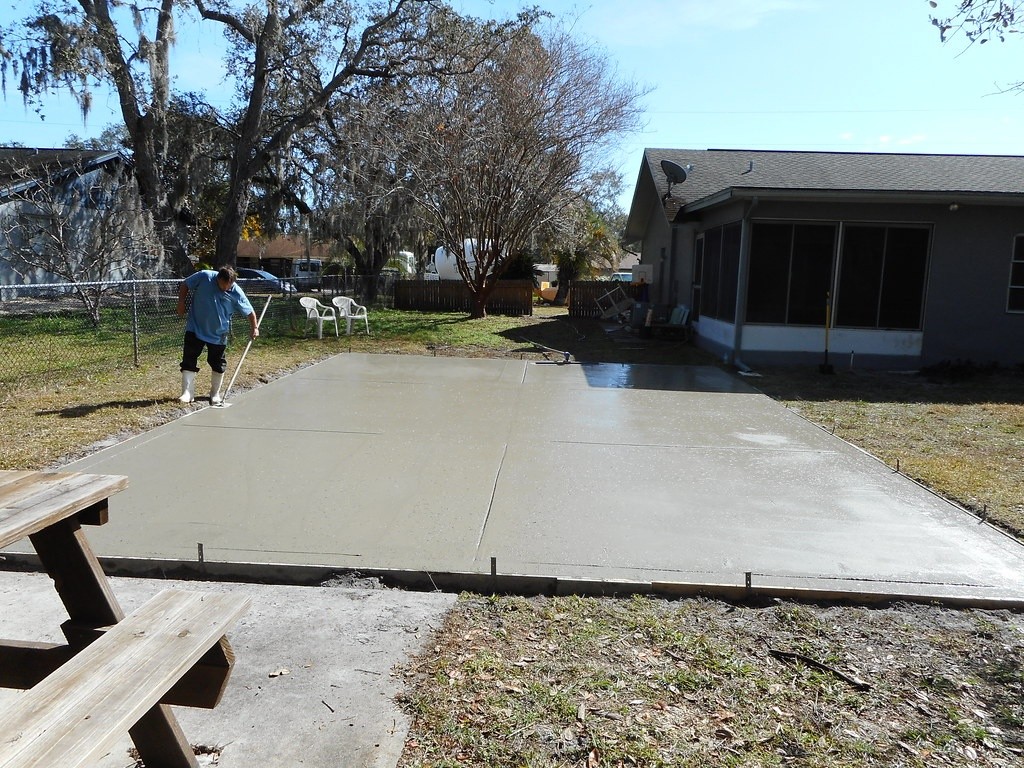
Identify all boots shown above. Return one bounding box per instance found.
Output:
[177,370,195,403]
[209,371,225,405]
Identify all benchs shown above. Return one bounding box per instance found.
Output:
[0,586,252,768]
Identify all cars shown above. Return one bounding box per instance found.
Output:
[607,273,632,282]
[235,267,297,293]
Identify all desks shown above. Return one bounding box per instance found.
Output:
[0,466,235,768]
[594,283,634,319]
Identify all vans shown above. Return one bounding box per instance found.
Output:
[291,253,322,292]
[380,251,415,279]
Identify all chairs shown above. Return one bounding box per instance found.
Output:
[332,295,370,336]
[299,297,339,340]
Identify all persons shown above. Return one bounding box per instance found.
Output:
[175,265,260,406]
